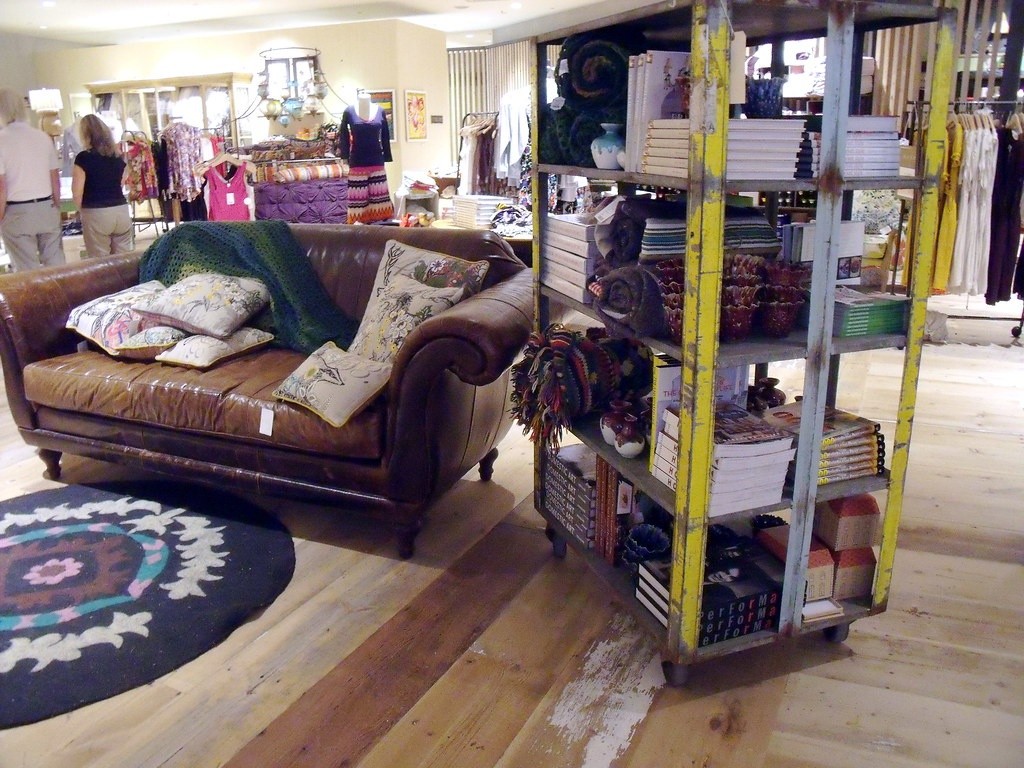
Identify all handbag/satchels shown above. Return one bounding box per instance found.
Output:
[924,310,949,343]
[895,103,924,201]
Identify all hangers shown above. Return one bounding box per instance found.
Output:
[921,101,1024,134]
[193,141,256,176]
[459,112,501,139]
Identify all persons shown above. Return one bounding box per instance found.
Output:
[339,93,393,224]
[72,114,135,258]
[0,86,68,271]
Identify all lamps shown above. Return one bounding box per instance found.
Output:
[255,46,330,127]
[29,87,64,138]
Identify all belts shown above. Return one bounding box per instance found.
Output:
[7,195,53,205]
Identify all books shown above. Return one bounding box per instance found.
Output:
[648,354,886,516]
[634,534,808,647]
[451,194,514,231]
[624,48,901,181]
[543,443,674,568]
[797,286,907,336]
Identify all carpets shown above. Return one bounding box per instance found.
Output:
[0,478,296,730]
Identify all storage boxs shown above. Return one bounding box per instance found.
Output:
[538,213,600,304]
[541,443,598,550]
[762,497,880,602]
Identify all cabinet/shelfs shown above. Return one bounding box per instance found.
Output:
[533,0,960,686]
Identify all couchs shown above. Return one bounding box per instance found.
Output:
[0,223,534,558]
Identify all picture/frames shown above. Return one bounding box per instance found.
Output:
[405,90,428,143]
[358,89,396,142]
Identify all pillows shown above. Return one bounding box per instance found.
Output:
[346,274,467,365]
[358,239,490,330]
[153,327,275,367]
[115,327,188,361]
[272,340,393,427]
[66,279,167,358]
[130,271,271,338]
